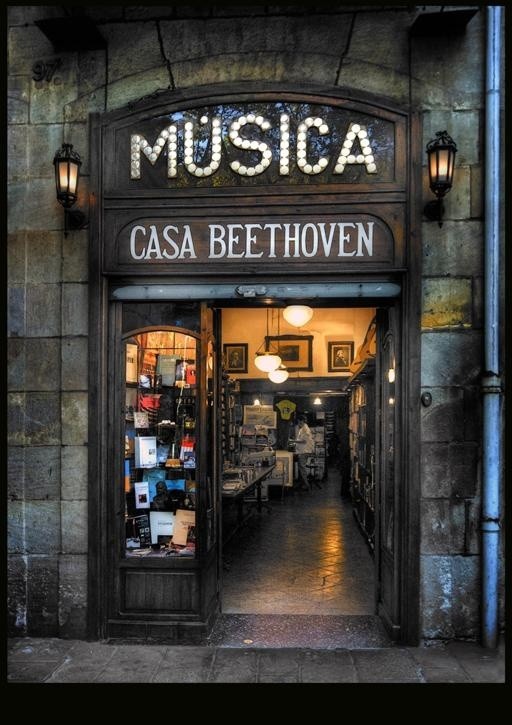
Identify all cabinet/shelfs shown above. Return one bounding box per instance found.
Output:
[343,358,375,562]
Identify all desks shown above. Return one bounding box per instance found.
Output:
[222,465,277,539]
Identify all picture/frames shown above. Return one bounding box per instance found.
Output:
[260,452,294,487]
[223,343,248,373]
[328,341,354,372]
[265,335,314,371]
[244,404,277,429]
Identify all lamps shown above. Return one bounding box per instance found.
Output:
[252,304,282,373]
[282,304,314,327]
[267,308,289,384]
[53,143,88,231]
[422,129,458,229]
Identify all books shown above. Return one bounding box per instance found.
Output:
[222,388,275,496]
[134,436,157,467]
[126,510,194,550]
[346,385,374,512]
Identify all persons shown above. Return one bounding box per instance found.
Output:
[288,415,314,495]
[154,482,166,507]
[334,348,346,366]
[229,351,241,367]
